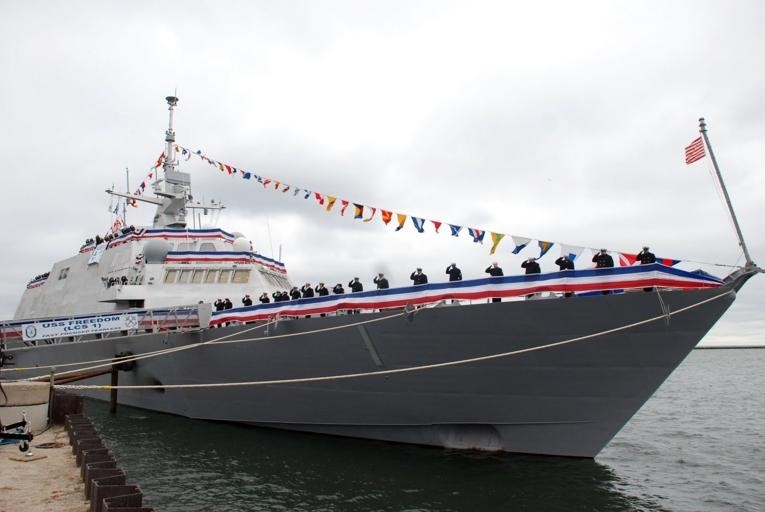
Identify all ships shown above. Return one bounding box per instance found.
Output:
[0,95,764,458]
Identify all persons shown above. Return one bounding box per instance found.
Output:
[591,248,615,294]
[120,275,128,286]
[32,272,50,283]
[636,245,657,292]
[444,262,463,304]
[484,261,504,302]
[111,277,120,285]
[78,225,136,255]
[520,255,541,299]
[212,273,363,329]
[555,255,575,296]
[107,277,114,287]
[372,272,390,313]
[410,267,427,309]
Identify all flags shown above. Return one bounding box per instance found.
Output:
[684,138,707,166]
[110,220,121,235]
